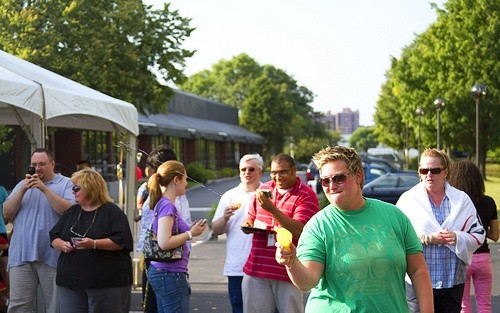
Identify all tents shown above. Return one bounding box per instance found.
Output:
[0,50,139,258]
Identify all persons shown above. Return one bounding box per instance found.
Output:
[212,152,274,313]
[3,148,78,313]
[440,158,500,313]
[396,149,483,313]
[75,159,91,171]
[0,185,10,291]
[240,154,318,313]
[48,168,134,313]
[137,145,207,313]
[275,146,435,313]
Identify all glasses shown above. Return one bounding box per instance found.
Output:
[418,167,446,174]
[240,167,260,172]
[70,216,94,238]
[319,171,356,186]
[72,185,82,192]
[271,167,291,176]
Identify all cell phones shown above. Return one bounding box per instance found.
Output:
[28,166,35,180]
[262,190,271,197]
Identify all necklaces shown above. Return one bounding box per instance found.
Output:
[70,209,97,239]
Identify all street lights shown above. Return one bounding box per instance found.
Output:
[415,107,425,166]
[470,85,486,172]
[434,98,448,150]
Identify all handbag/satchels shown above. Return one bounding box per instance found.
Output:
[142,200,183,262]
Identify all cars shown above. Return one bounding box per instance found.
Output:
[361,172,422,205]
[305,147,404,195]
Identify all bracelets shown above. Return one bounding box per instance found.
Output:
[94,239,97,249]
[187,230,192,239]
[287,259,295,270]
